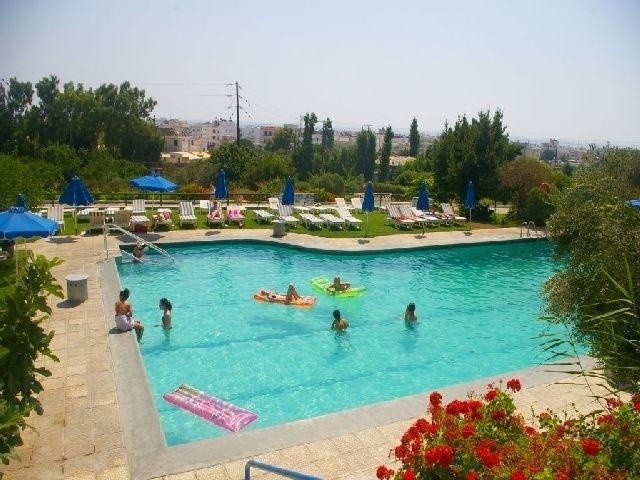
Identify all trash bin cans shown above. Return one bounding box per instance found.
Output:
[272,220,286,236]
[65,274,89,302]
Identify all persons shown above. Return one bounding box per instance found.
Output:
[327,276,351,292]
[115,288,143,344]
[153,212,165,230]
[261,283,302,302]
[331,310,349,329]
[406,303,417,320]
[210,201,219,220]
[135,241,142,256]
[159,298,172,328]
[209,181,243,206]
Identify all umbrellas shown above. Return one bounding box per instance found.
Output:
[464,181,476,225]
[59,175,94,229]
[128,173,177,214]
[362,181,375,231]
[417,182,429,230]
[282,176,294,217]
[0,194,57,285]
[215,169,228,209]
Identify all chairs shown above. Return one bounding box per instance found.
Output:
[176,200,248,229]
[34,198,172,236]
[375,197,469,235]
[253,198,366,231]
